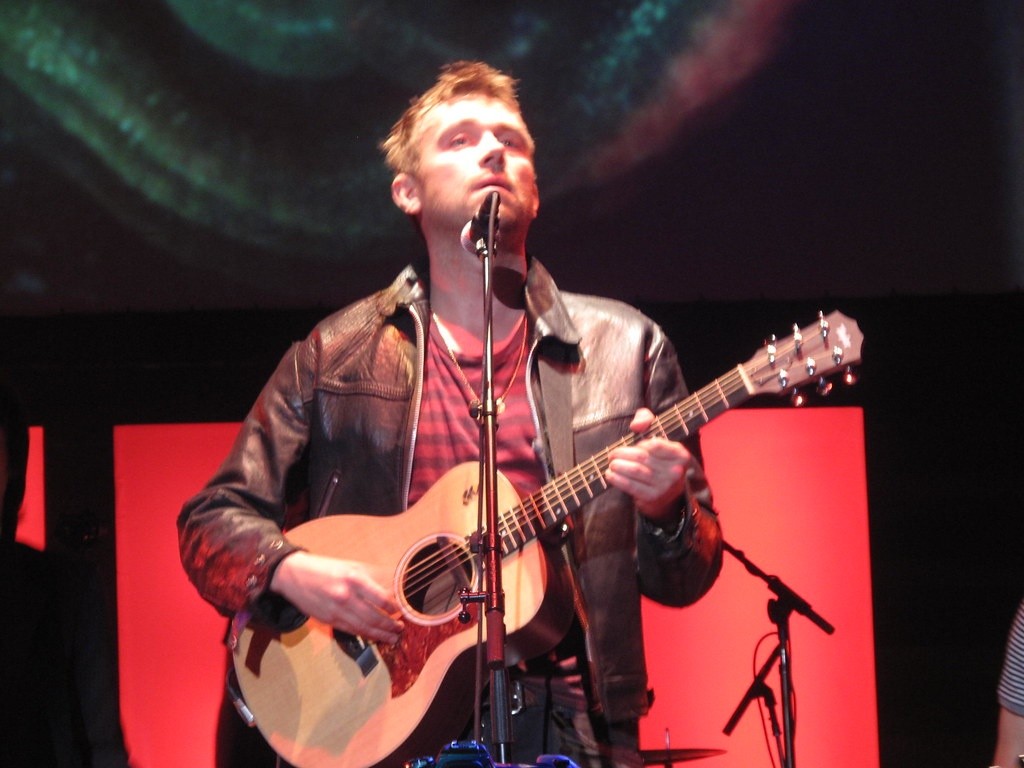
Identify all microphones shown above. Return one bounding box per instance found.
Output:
[460,191,501,256]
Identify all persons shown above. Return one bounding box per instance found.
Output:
[990,596,1024,768]
[176,59,724,767]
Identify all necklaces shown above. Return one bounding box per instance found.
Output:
[432,310,527,416]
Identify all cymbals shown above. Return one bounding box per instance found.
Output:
[640,748,730,764]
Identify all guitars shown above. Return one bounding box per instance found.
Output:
[234,310,866,767]
